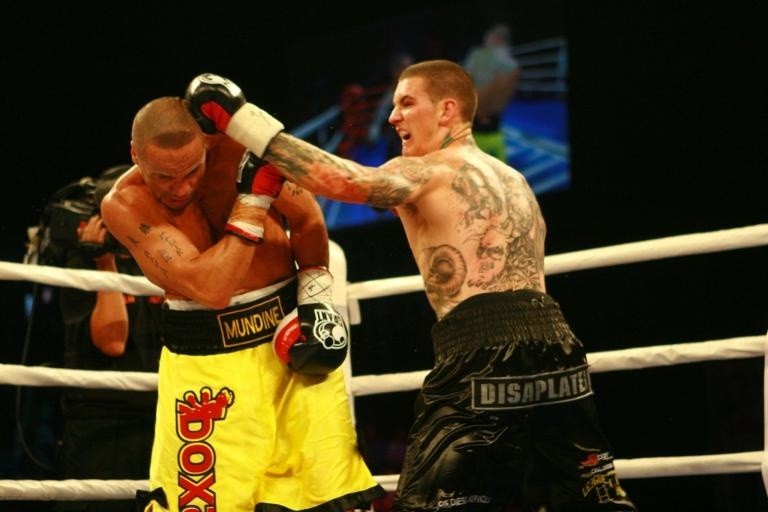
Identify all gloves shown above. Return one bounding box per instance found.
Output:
[223,149,286,246]
[184,71,286,160]
[271,265,349,377]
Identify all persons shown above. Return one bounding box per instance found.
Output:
[186,58,637,511]
[365,52,416,163]
[458,19,521,163]
[98,95,387,511]
[332,68,390,160]
[23,164,166,511]
